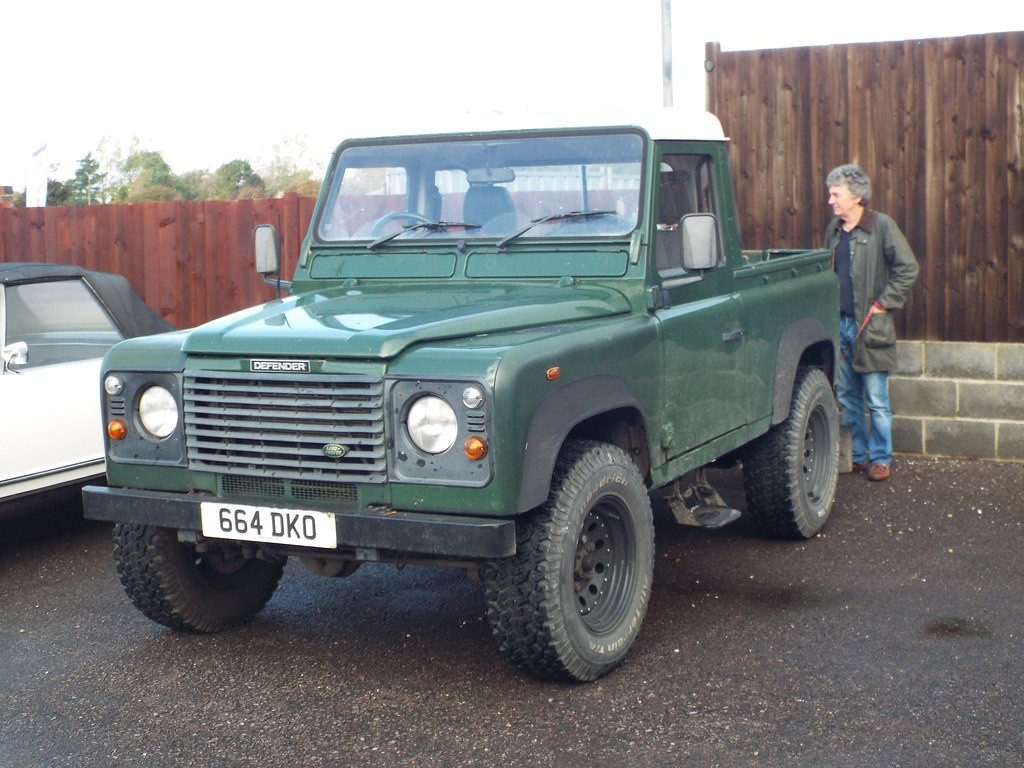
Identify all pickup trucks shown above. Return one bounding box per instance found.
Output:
[81,106,843,685]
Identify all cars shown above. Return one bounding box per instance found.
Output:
[0,262,177,520]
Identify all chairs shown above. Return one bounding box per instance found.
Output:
[621,171,699,271]
[462,186,518,237]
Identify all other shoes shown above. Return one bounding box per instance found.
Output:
[853,460,866,471]
[866,464,890,480]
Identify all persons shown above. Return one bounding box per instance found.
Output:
[823,163,920,481]
